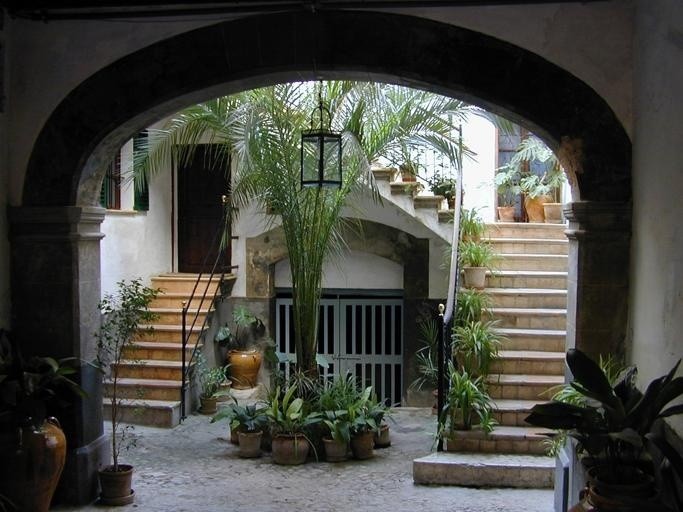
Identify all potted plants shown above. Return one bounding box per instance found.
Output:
[451,319,511,377]
[441,205,497,243]
[440,242,507,291]
[386,153,428,182]
[212,391,269,458]
[416,354,500,452]
[188,351,236,414]
[269,388,394,467]
[93,276,167,505]
[452,284,494,327]
[491,130,564,224]
[301,80,342,192]
[522,345,683,512]
[0,332,104,512]
[214,304,280,389]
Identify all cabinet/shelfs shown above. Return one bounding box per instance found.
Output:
[553,433,587,511]
